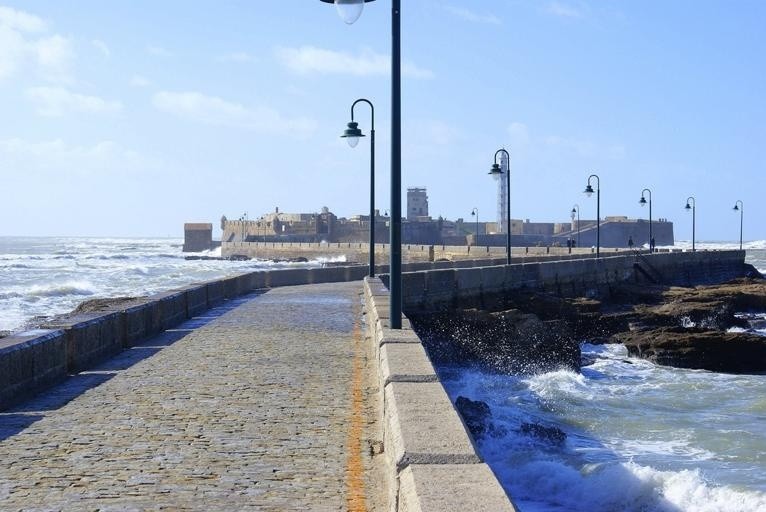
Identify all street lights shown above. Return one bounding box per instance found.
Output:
[470,207,478,246]
[487,149,511,267]
[732,198,742,250]
[639,188,652,253]
[322,0,401,335]
[581,173,600,259]
[569,203,580,248]
[684,195,695,252]
[338,96,375,278]
